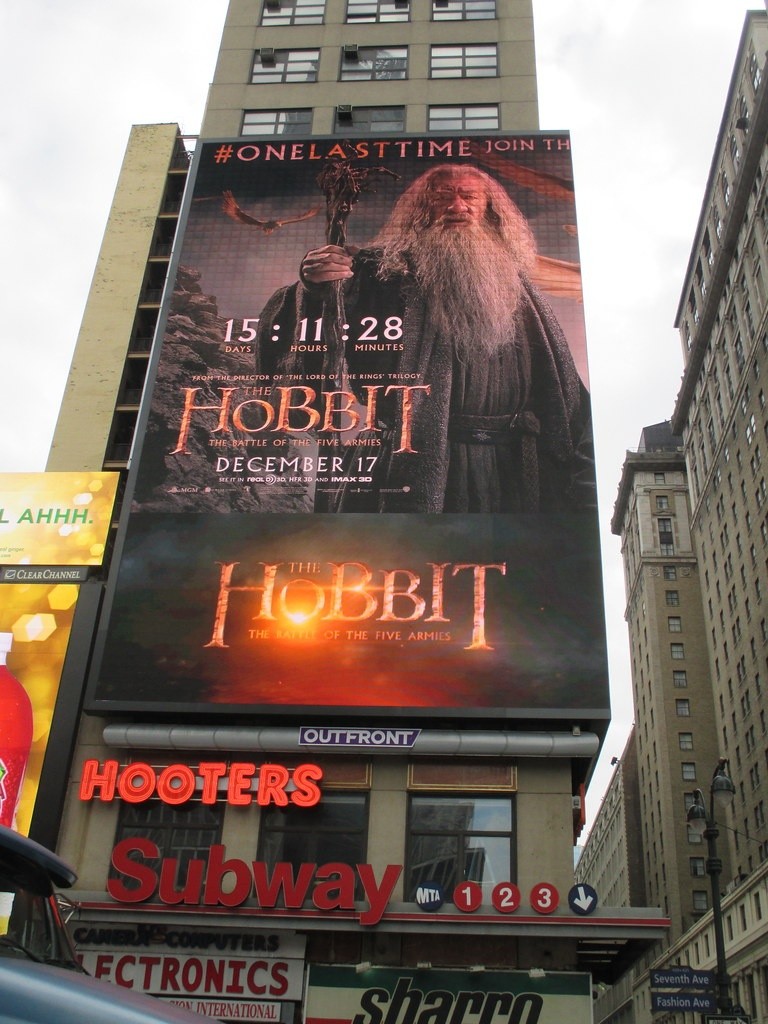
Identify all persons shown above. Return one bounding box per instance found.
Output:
[253,163,598,517]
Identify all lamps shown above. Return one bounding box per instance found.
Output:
[529,968,545,979]
[356,959,371,972]
[417,961,431,968]
[470,961,485,972]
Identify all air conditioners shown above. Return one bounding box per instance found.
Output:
[266,0,280,8]
[337,104,352,113]
[260,47,274,60]
[343,43,357,52]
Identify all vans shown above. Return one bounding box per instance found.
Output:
[0,824,226,1024]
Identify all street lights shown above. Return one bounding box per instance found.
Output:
[685,755,749,1024]
